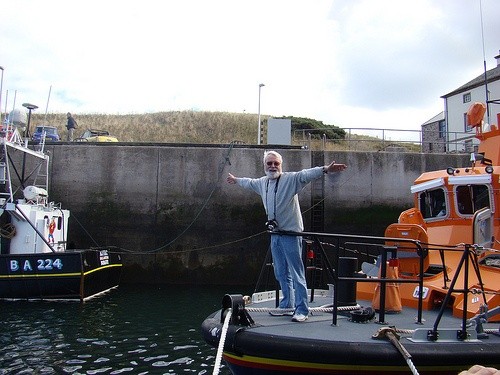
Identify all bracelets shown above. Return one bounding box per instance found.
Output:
[323,167,328,174]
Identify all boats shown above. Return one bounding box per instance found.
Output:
[202,101,499,375]
[0,100,125,304]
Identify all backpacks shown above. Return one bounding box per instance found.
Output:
[74,121,78,129]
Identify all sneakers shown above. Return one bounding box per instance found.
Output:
[292,313,308,322]
[268,307,294,315]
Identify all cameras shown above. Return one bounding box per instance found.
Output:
[265,219,279,230]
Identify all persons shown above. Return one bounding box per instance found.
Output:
[226,151,348,323]
[64,111,78,142]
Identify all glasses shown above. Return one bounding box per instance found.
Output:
[267,162,281,166]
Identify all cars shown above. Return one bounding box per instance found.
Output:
[80,129,120,142]
[31,125,61,142]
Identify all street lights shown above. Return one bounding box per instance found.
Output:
[256,83,265,143]
